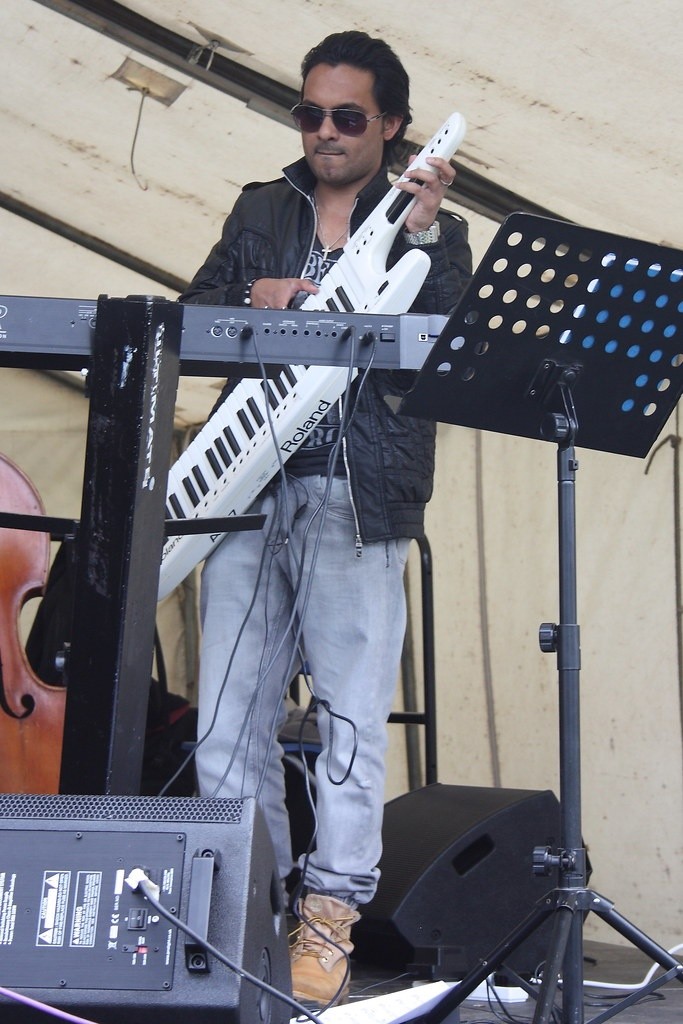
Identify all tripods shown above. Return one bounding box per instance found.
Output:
[385,210,683,1024]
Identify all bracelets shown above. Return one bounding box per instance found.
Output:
[403,220,441,245]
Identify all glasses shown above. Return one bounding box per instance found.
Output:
[291,101,388,138]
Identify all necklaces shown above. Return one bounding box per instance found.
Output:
[314,189,348,261]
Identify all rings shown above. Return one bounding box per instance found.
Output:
[439,175,453,186]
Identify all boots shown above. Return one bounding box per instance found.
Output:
[289,893,362,1004]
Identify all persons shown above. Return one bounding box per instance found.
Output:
[173,31,477,1006]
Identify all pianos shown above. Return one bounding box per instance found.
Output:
[153,108,473,604]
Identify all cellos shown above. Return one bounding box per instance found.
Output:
[0,448,72,793]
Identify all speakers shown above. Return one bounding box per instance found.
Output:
[350,783,591,986]
[0,792,295,1024]
